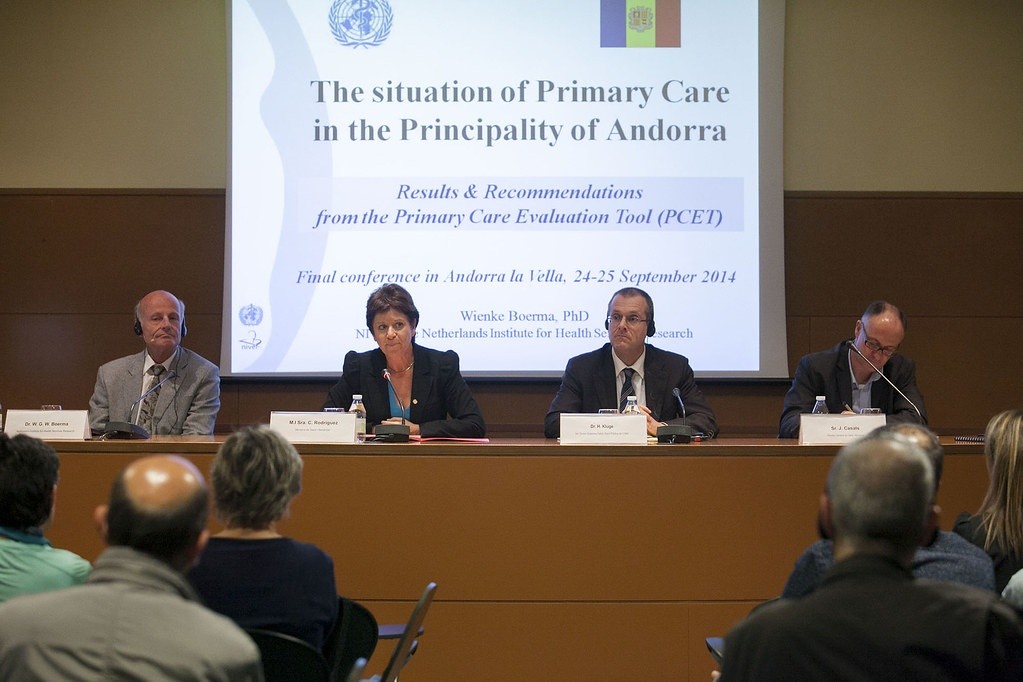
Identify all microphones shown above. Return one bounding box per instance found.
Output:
[656,388,691,443]
[104,369,176,440]
[844,340,922,425]
[374,369,410,441]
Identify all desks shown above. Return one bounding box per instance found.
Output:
[35,432,989,682]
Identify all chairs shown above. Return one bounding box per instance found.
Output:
[376,581,439,682]
[238,623,367,682]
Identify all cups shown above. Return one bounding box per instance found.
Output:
[860,408,881,415]
[323,407,345,412]
[41,405,62,411]
[598,409,619,415]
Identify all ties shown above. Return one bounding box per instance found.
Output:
[138,365,165,433]
[619,368,636,414]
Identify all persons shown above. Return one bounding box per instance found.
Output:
[87,290,220,436]
[320,282,486,439]
[776,300,926,437]
[181,425,378,682]
[0,456,262,682]
[710,409,1023,682]
[0,431,93,607]
[543,287,719,439]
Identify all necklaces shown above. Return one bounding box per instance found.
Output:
[387,362,414,372]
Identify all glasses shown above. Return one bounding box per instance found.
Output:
[861,321,900,356]
[607,314,648,325]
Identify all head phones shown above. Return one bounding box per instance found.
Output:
[605,312,655,336]
[134,313,187,338]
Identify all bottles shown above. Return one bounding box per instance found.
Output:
[622,395,641,415]
[811,396,830,415]
[348,394,367,444]
